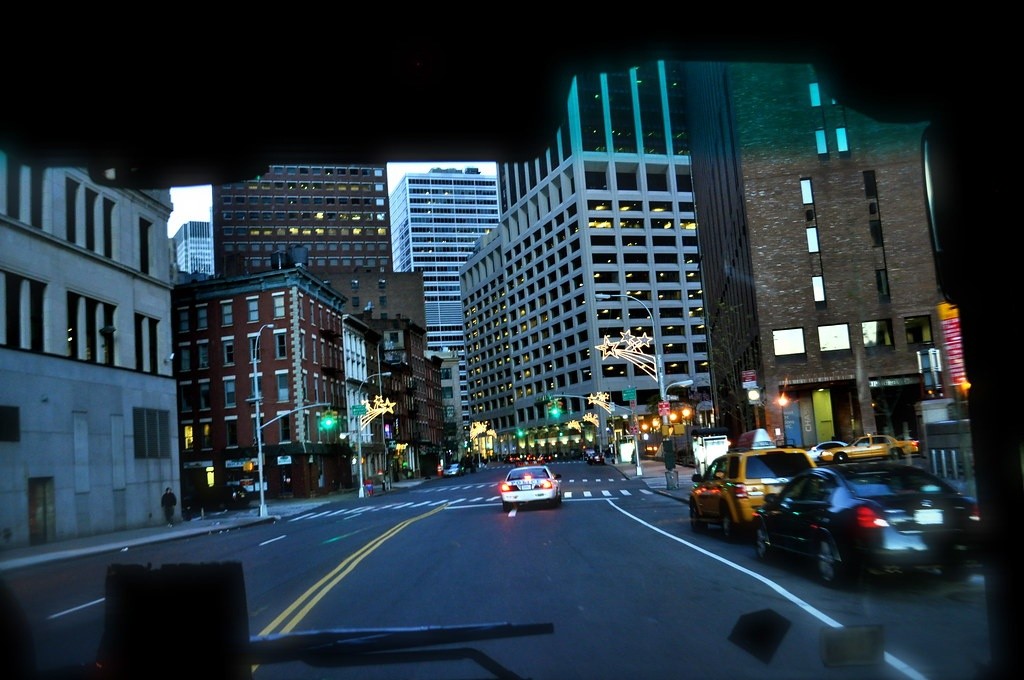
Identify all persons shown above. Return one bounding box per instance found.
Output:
[162,487,176,528]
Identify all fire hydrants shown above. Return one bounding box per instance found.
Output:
[381,480,386,491]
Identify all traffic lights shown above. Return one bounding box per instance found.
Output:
[517,431,524,437]
[548,399,562,416]
[322,410,338,429]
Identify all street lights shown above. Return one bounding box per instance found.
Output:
[358,372,391,498]
[377,339,389,443]
[550,393,643,476]
[254,324,330,518]
[595,293,694,490]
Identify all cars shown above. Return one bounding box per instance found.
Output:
[522,454,537,462]
[752,461,984,594]
[538,453,553,463]
[818,433,921,464]
[501,465,562,512]
[689,428,818,544]
[443,463,465,477]
[181,486,249,511]
[807,441,849,466]
[587,453,603,466]
[504,454,524,463]
[603,448,637,464]
[676,446,693,466]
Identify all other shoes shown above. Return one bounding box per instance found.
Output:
[167,523,173,528]
